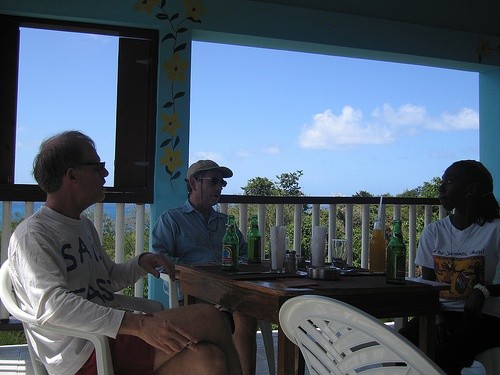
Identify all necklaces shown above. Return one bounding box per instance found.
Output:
[449,215,475,270]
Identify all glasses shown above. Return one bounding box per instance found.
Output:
[76,162,105,169]
[437,179,458,185]
[202,176,227,187]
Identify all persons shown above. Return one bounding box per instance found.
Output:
[151,160,258,375]
[7,131,243,375]
[395,160,500,375]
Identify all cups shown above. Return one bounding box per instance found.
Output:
[269,238,287,273]
[332,239,348,271]
[310,238,325,268]
[284,249,297,273]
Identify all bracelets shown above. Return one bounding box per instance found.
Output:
[473,283,490,300]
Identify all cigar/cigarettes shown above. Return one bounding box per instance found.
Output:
[185,340,193,348]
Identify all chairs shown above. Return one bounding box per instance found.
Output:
[159,272,275,375]
[278,294,448,375]
[394,317,500,375]
[0,258,162,375]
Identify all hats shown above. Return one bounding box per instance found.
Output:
[186,160,233,180]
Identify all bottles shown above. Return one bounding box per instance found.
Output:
[247,215,262,264]
[386,220,406,284]
[220,215,240,271]
[368,218,387,272]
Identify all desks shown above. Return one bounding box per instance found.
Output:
[174,260,452,375]
